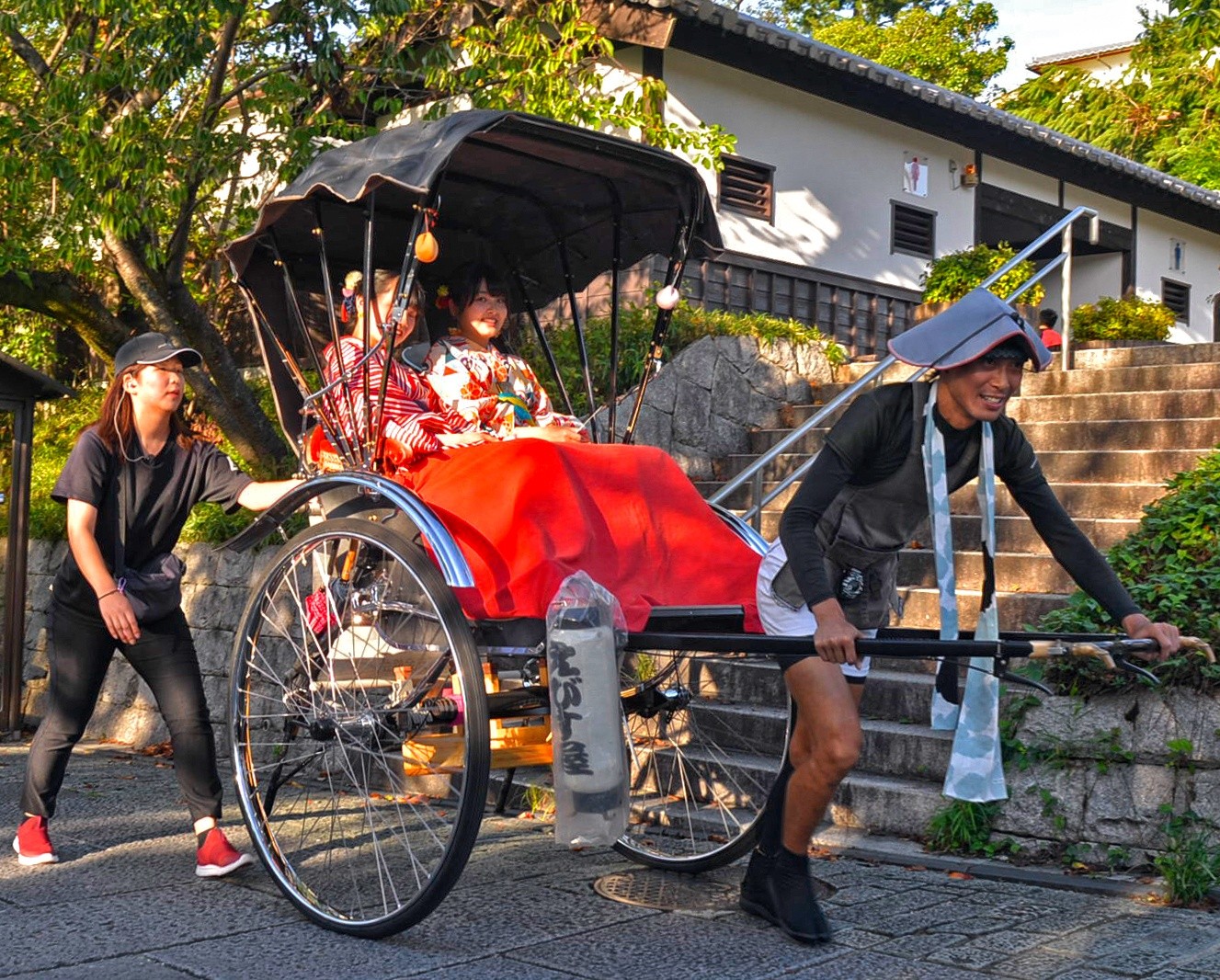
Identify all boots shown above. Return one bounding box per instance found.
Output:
[767,847,832,942]
[738,848,787,925]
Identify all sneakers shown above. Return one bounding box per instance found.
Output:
[193,831,257,878]
[13,815,59,864]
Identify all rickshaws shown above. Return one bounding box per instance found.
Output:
[211,110,1212,939]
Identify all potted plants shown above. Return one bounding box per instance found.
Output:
[912,240,1046,339]
[1069,293,1179,351]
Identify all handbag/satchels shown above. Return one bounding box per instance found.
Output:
[112,553,185,621]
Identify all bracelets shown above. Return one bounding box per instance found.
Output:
[98,590,120,601]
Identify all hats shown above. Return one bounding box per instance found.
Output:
[115,333,204,377]
[887,287,1052,371]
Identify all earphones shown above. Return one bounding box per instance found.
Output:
[127,383,133,387]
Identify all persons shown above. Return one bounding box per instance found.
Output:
[424,265,590,443]
[13,331,319,878]
[320,268,499,452]
[1039,308,1062,352]
[738,284,1180,938]
